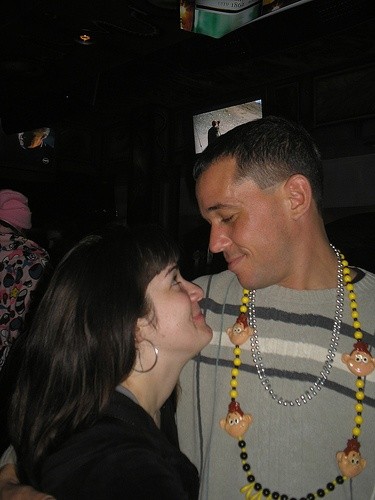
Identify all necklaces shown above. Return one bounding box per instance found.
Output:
[220,243,375,500]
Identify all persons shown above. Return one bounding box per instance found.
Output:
[0,232,214,500]
[208,120,222,146]
[0,189,49,370]
[0,115,375,500]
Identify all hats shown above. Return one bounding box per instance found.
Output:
[0,189,32,229]
